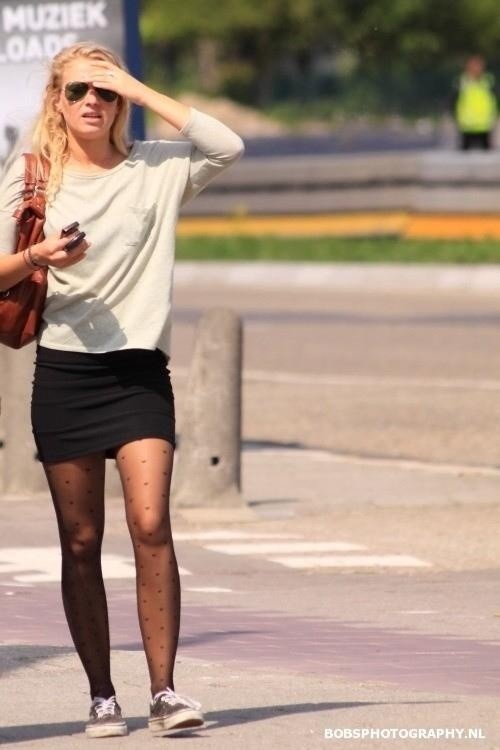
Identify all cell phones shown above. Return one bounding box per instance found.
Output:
[60,221,80,239]
[64,232,86,251]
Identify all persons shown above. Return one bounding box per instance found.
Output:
[446,54,500,151]
[0,44,245,738]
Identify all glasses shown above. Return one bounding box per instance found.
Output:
[63,81,119,105]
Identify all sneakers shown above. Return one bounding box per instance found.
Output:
[84,693,128,738]
[148,691,204,737]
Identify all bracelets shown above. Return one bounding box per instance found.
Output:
[17,248,40,278]
[27,243,46,273]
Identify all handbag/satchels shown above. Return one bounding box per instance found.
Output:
[1,192,47,350]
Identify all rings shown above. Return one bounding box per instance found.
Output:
[108,68,115,77]
